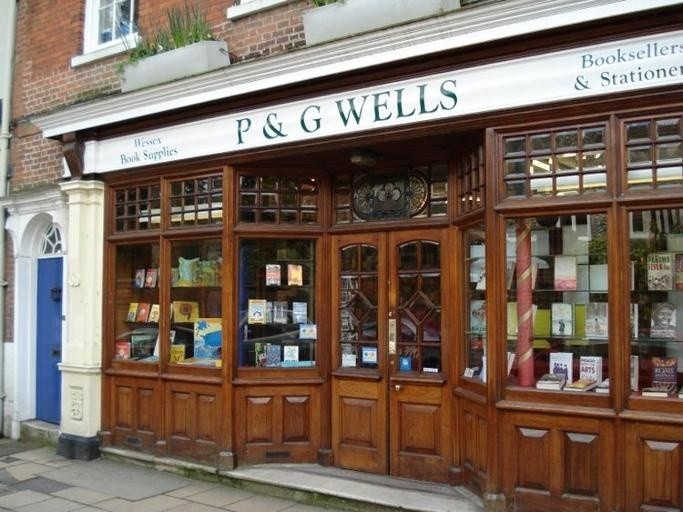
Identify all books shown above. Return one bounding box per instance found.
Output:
[111,258,317,371]
[468,251,682,400]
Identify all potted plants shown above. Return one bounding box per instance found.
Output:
[112,1,232,96]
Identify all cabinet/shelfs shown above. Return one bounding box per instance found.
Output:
[450,205,683,400]
[237,238,317,368]
[123,276,224,333]
